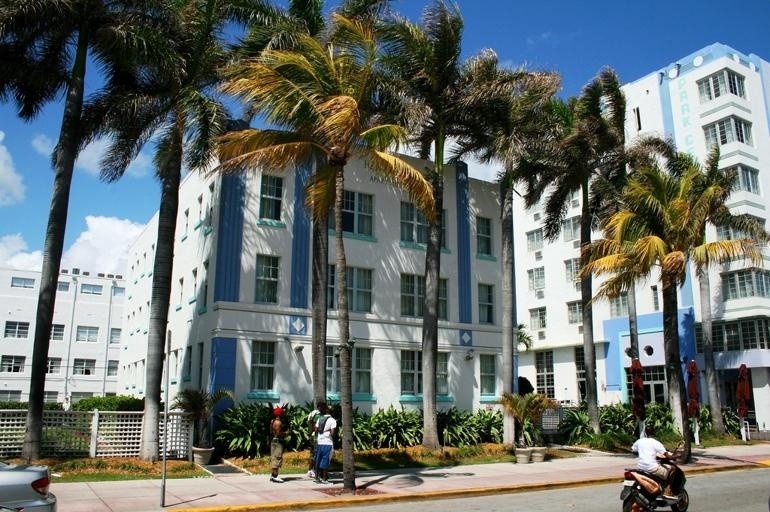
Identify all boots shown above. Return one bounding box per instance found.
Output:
[662,483,680,500]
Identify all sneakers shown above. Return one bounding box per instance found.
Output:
[269,469,330,484]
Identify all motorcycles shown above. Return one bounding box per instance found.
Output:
[616,436,691,511]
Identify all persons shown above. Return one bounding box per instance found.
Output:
[307,402,323,479]
[270,408,290,483]
[313,405,337,482]
[631,426,679,500]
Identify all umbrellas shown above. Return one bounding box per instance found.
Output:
[630,355,649,440]
[687,360,700,446]
[736,363,750,441]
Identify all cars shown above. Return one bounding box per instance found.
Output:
[0,457,58,512]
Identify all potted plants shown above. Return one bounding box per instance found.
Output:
[529,391,562,462]
[494,389,540,465]
[168,384,239,466]
